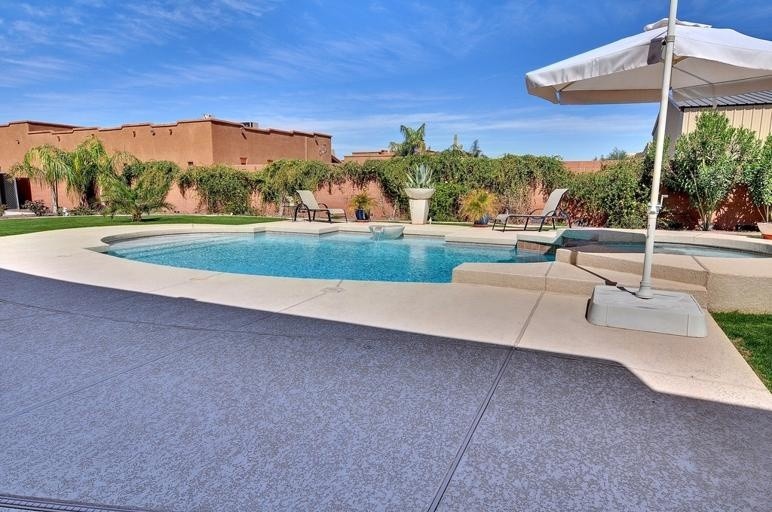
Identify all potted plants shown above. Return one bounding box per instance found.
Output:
[404,162,435,199]
[741,131,772,239]
[346,188,378,222]
[456,188,500,227]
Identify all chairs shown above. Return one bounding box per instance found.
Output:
[491,188,571,232]
[293,190,347,223]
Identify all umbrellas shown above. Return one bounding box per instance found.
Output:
[521,15,772,301]
